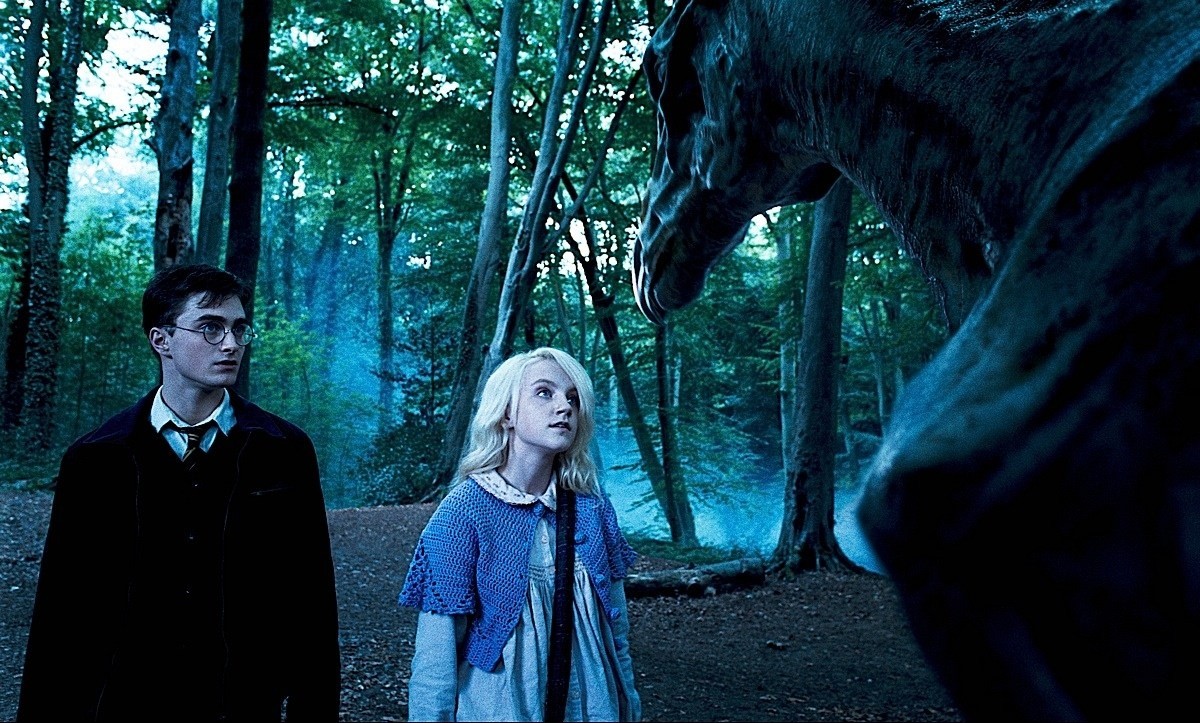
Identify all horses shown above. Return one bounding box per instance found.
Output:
[619,1,1200,723]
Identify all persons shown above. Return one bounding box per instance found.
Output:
[399,347,642,722]
[14,262,340,723]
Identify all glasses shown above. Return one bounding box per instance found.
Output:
[162,320,254,347]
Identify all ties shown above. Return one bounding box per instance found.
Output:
[181,426,208,472]
